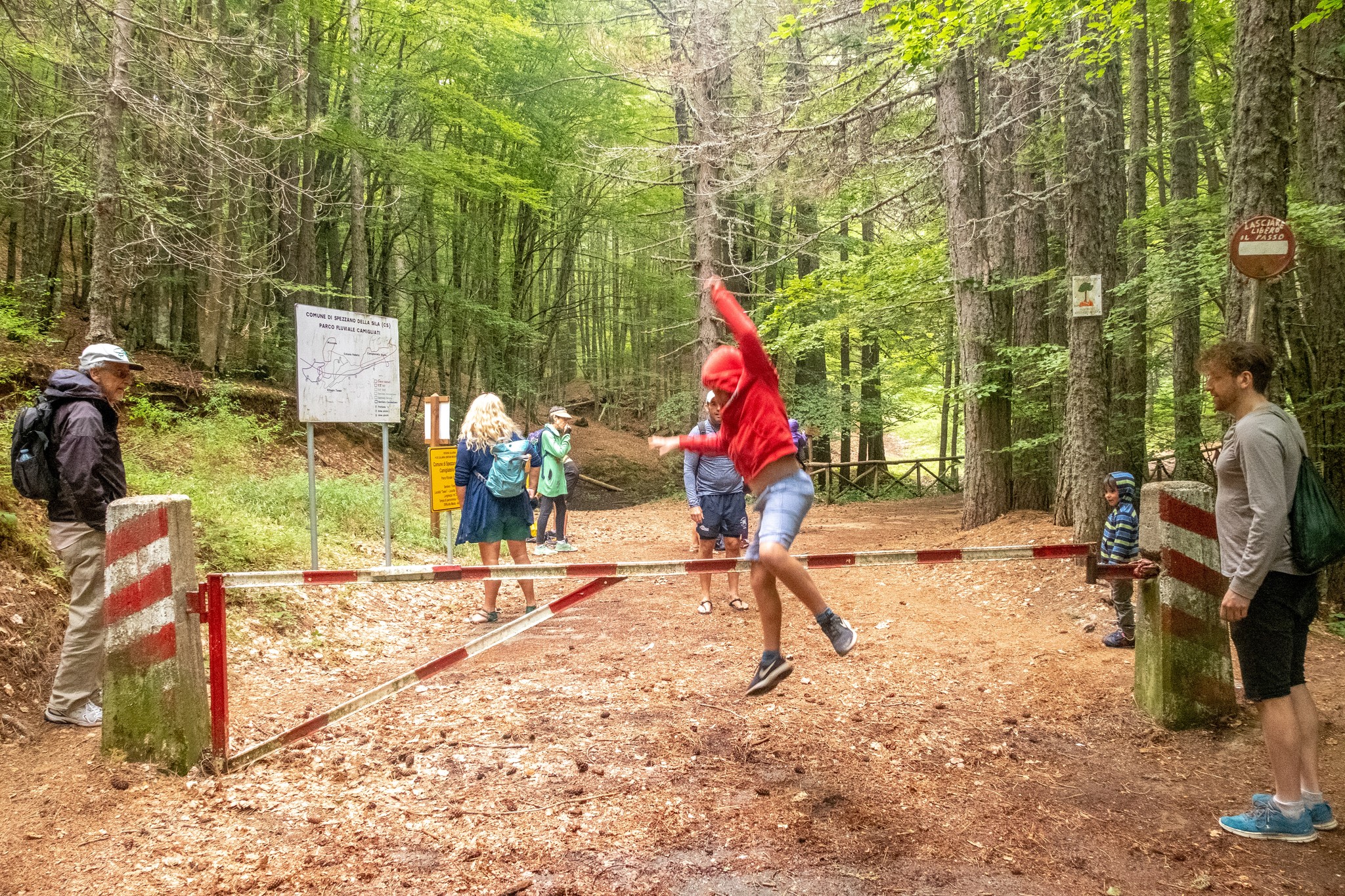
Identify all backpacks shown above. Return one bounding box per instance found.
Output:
[526,428,556,466]
[471,435,530,498]
[9,394,97,500]
[1245,409,1345,573]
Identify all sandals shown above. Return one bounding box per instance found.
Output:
[526,603,542,615]
[462,608,498,625]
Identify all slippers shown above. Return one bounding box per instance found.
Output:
[697,598,713,615]
[729,598,749,611]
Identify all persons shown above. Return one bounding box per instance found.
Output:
[533,406,578,556]
[1100,471,1139,649]
[1196,339,1338,844]
[526,472,539,543]
[454,391,542,624]
[647,273,857,697]
[788,419,807,466]
[530,454,579,547]
[44,343,145,727]
[683,390,749,614]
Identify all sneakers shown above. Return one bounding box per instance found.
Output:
[555,542,578,551]
[1101,629,1135,648]
[533,544,558,555]
[1252,793,1338,830]
[1216,795,1318,843]
[743,655,793,698]
[88,688,104,707]
[544,530,557,538]
[43,696,102,727]
[545,537,568,545]
[818,611,858,656]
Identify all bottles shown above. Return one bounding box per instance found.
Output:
[19,449,33,462]
[525,454,532,473]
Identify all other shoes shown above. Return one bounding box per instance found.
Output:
[740,538,750,549]
[689,544,719,555]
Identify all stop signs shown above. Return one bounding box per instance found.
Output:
[1229,216,1296,280]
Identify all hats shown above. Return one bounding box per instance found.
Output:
[550,410,573,419]
[77,343,145,371]
[705,390,716,404]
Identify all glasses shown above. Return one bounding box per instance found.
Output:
[106,368,127,379]
